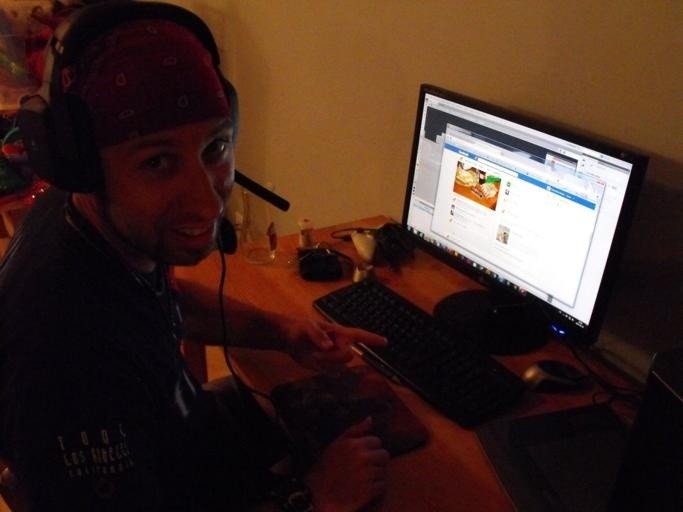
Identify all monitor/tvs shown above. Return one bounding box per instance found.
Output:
[400,84,650,356]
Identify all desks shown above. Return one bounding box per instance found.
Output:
[169,210,682,512]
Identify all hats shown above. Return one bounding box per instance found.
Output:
[74,21,233,153]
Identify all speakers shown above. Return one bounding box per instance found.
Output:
[607,351,683,512]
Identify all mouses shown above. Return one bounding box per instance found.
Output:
[521,358,594,397]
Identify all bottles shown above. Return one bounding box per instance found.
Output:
[294,216,322,260]
[239,180,279,266]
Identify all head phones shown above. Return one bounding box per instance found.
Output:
[15,0,239,195]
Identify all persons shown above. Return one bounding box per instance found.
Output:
[23,0,72,87]
[0,0,398,512]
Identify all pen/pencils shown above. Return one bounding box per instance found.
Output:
[349,344,402,386]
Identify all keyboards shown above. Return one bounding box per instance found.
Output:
[312,279,528,430]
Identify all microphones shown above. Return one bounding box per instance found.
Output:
[216,216,237,253]
